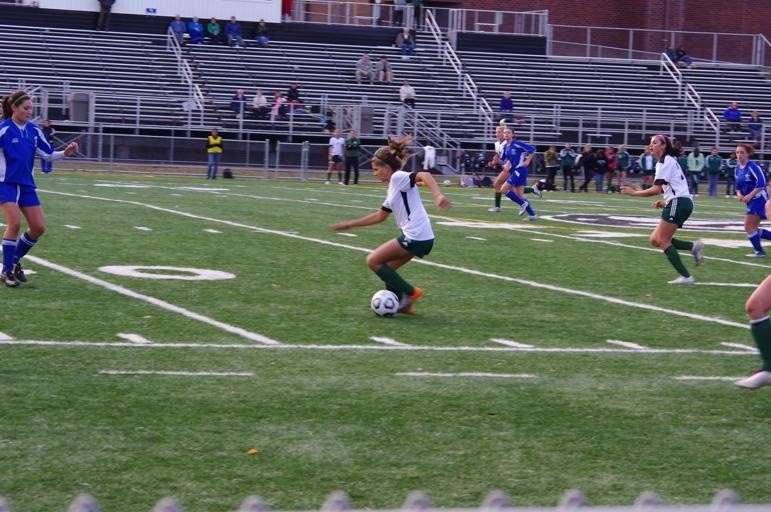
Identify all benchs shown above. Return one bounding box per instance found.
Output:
[0,24,770,187]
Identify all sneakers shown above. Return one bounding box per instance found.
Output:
[542,190,554,192]
[724,195,737,198]
[399,287,422,314]
[692,241,704,266]
[667,275,694,284]
[734,369,771,389]
[528,214,537,220]
[325,181,330,184]
[518,201,528,216]
[338,181,343,184]
[532,185,542,198]
[0,270,19,287]
[745,251,766,258]
[13,263,26,282]
[488,207,501,212]
[690,193,699,198]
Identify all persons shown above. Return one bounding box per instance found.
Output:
[543,143,738,198]
[735,274,770,390]
[621,134,705,284]
[230,83,304,117]
[0,91,78,286]
[489,123,537,216]
[748,111,763,139]
[40,120,56,173]
[96,0,115,30]
[331,135,452,315]
[724,102,741,131]
[733,143,770,258]
[395,27,415,60]
[207,128,223,179]
[355,54,393,85]
[172,15,246,47]
[326,128,360,186]
[255,20,269,48]
[495,91,516,124]
[666,46,695,70]
[399,80,416,108]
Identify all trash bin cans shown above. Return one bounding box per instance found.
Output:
[353,106,373,134]
[68,91,89,121]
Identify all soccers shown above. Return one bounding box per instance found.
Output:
[372,289,398,318]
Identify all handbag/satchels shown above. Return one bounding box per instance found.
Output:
[223,169,232,179]
